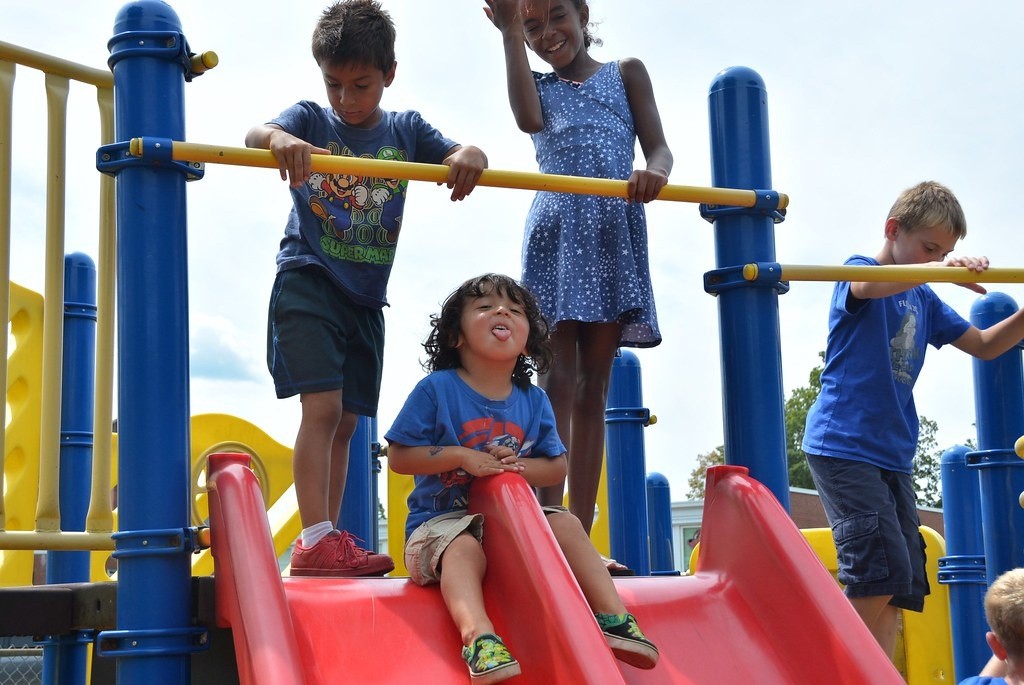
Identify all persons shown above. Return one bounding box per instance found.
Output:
[245,0,487,578]
[480,0,674,578]
[801,180,1024,666]
[957,565,1024,685]
[383,273,660,685]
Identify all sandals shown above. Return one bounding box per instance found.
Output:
[599,553,636,576]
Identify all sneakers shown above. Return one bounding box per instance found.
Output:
[593,611,659,670]
[462,632,521,685]
[290,529,395,577]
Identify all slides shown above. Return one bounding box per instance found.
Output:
[205,447,906,685]
